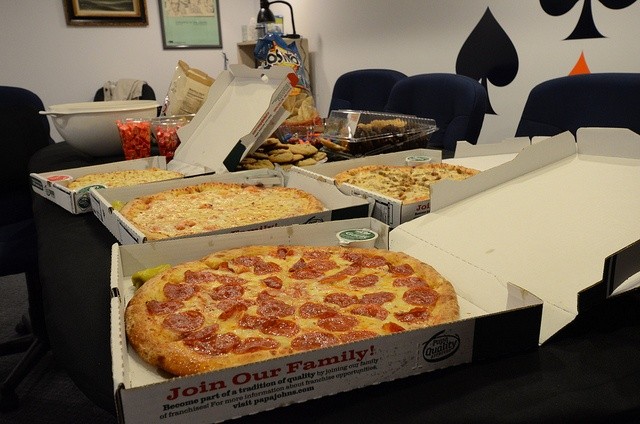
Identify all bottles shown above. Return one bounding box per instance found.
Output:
[256,1,275,53]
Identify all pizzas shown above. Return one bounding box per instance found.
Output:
[121,180,323,243]
[68,168,184,192]
[332,162,482,205]
[125,244,461,375]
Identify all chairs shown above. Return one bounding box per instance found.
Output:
[514,72,639,136]
[328,68,409,120]
[383,73,488,159]
[0,86,56,186]
[93,79,156,102]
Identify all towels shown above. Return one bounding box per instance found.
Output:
[104,81,147,101]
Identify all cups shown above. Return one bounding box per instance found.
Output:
[76,183,107,194]
[242,25,246,42]
[152,113,196,164]
[406,156,431,166]
[46,175,73,187]
[114,118,151,160]
[336,228,378,248]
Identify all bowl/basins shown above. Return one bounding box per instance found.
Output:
[39,100,159,158]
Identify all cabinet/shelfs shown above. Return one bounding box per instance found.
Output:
[236,38,311,82]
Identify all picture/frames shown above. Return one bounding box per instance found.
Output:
[158,0,223,49]
[62,0,149,27]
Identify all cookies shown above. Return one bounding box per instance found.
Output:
[270,154,292,162]
[290,153,304,162]
[244,160,275,170]
[312,151,327,161]
[289,143,318,156]
[295,157,318,167]
[241,157,257,164]
[252,151,269,159]
[269,148,290,155]
[262,138,279,146]
[270,144,290,149]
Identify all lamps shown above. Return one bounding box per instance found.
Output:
[257,0,301,38]
